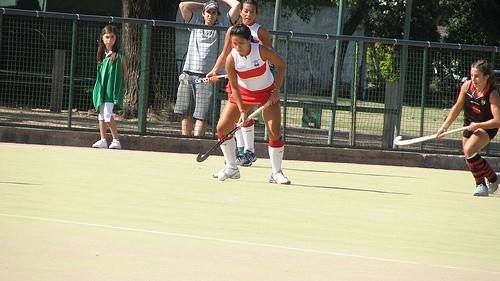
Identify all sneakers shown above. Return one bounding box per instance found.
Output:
[240,149,257,166]
[269,170,291,184]
[108,140,121,148]
[92,140,107,148]
[473,184,488,196]
[224,151,244,165]
[486,173,500,193]
[213,165,240,179]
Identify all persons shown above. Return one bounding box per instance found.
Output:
[174,0,240,137]
[206,0,293,185]
[434,61,500,196]
[91,25,128,149]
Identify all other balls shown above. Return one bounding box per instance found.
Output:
[217,172,226,181]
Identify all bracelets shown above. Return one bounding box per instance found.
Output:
[240,110,247,114]
[274,88,280,91]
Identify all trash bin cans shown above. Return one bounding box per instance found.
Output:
[302,106,322,128]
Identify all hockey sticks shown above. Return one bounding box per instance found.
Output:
[179,73,228,85]
[196,100,272,162]
[393,125,470,146]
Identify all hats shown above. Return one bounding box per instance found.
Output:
[202,1,219,12]
